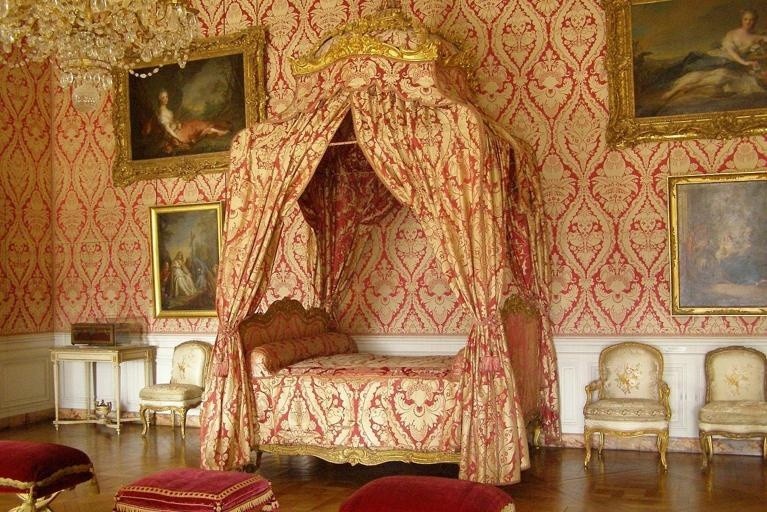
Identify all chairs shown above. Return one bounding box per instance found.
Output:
[696,343,765,475]
[582,340,671,473]
[137,339,212,442]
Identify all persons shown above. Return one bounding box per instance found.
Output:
[651,10,766,105]
[158,251,209,297]
[141,89,230,152]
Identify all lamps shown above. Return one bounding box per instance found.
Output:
[0,0,203,116]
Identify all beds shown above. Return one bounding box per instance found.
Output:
[197,6,574,487]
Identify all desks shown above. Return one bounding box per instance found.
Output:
[45,339,160,441]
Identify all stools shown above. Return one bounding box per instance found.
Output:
[112,462,279,511]
[331,470,522,512]
[1,428,103,511]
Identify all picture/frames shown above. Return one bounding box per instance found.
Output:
[600,0,767,153]
[145,197,225,321]
[111,23,268,190]
[665,170,766,318]
